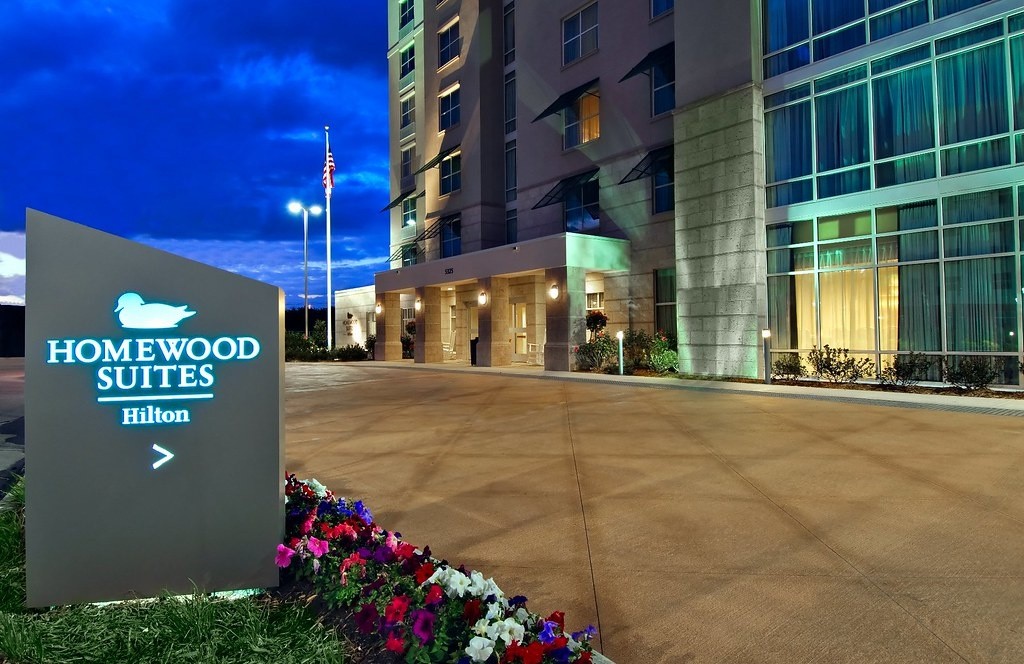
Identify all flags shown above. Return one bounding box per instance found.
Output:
[322,144,335,187]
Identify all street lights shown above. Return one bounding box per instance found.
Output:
[288,202,322,339]
